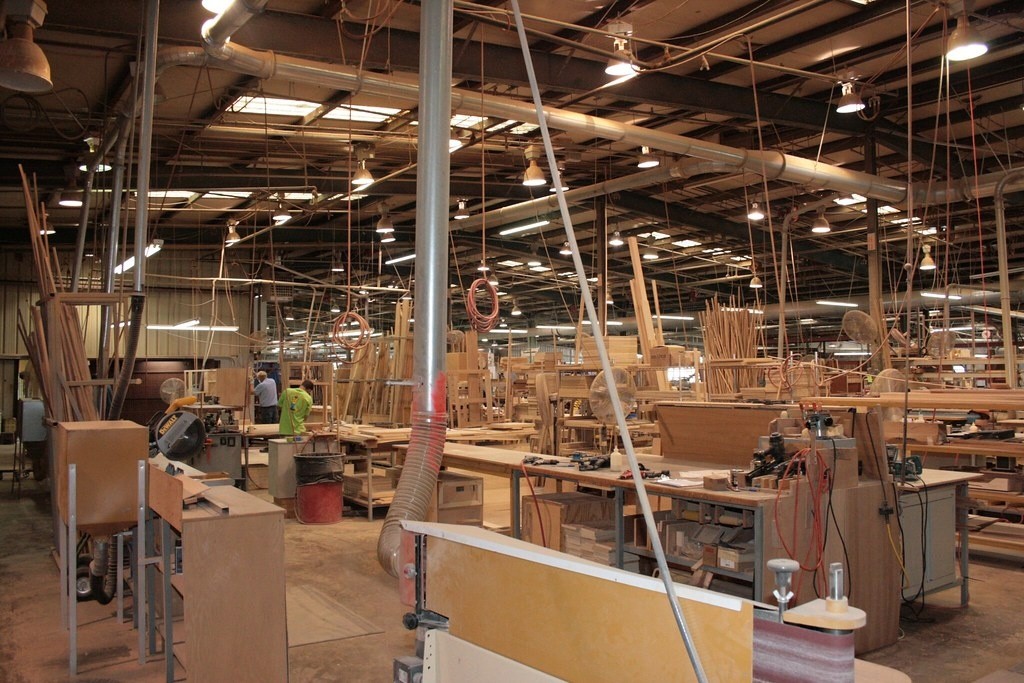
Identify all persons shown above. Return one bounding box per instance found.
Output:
[248,371,279,452]
[277,379,314,439]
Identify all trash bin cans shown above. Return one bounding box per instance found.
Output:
[292,451,347,525]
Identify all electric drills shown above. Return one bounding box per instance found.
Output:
[752,432,785,462]
[522,455,560,466]
[619,469,654,480]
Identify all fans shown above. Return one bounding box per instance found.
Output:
[869,368,906,421]
[589,367,637,496]
[891,328,907,346]
[156,411,206,461]
[928,331,955,359]
[842,310,879,346]
[160,378,185,406]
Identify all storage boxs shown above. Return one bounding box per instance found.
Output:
[436,471,484,524]
[883,421,947,445]
[670,350,701,367]
[704,543,718,566]
[717,542,755,573]
[520,492,616,554]
[649,344,683,367]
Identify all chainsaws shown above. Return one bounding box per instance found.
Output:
[148,395,236,486]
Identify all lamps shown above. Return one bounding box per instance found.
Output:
[750,277,763,288]
[522,145,546,185]
[811,206,831,233]
[109,318,239,332]
[39,222,56,236]
[489,267,499,285]
[454,202,470,220]
[59,169,83,206]
[643,236,658,260]
[78,134,112,172]
[331,300,340,313]
[286,308,294,320]
[549,162,570,192]
[497,216,550,235]
[224,218,240,243]
[478,258,490,271]
[638,144,659,169]
[747,200,764,220]
[385,250,416,265]
[945,0,988,61]
[559,239,572,255]
[376,203,395,234]
[380,232,396,242]
[607,289,614,305]
[604,23,639,76]
[273,193,293,221]
[835,82,866,114]
[499,318,507,328]
[351,142,375,185]
[919,244,936,271]
[115,238,164,275]
[511,298,522,315]
[609,230,624,247]
[0,0,53,93]
[332,250,344,272]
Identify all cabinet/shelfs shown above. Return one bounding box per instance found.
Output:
[16,332,1024,683]
